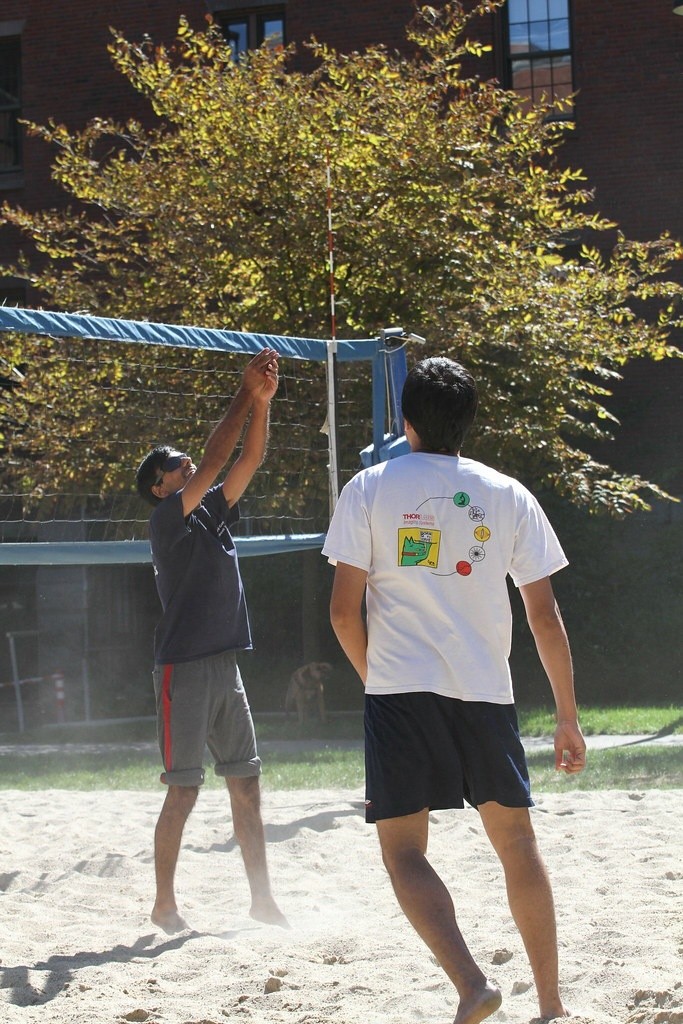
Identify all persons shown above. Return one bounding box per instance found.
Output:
[321,358,586,1023]
[137,347,288,934]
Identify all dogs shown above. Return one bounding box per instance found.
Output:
[285,661,334,727]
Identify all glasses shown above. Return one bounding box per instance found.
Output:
[154,452,188,487]
